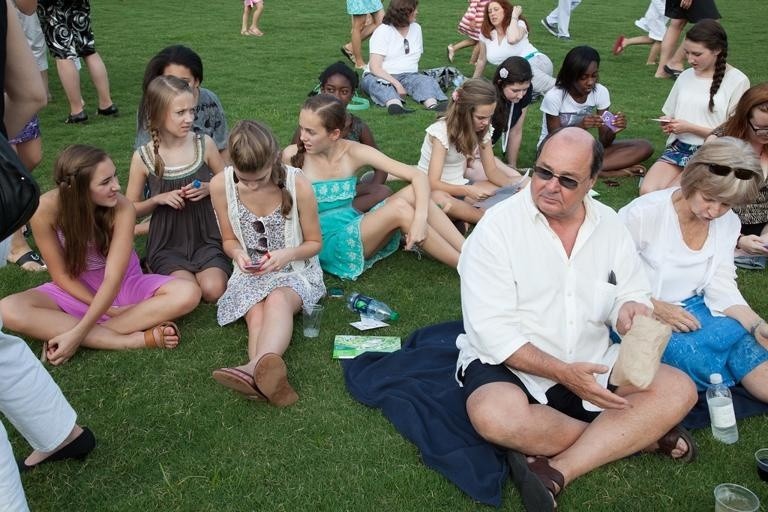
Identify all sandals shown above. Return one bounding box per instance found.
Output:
[341,44,356,64]
[655,424,697,463]
[506,448,564,512]
[247,26,262,37]
[240,31,250,35]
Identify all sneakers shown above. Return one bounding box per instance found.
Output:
[541,17,559,37]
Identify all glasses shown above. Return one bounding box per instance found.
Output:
[534,166,592,190]
[697,162,755,180]
[747,119,768,136]
[252,220,267,248]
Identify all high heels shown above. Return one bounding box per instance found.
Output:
[17,426,96,472]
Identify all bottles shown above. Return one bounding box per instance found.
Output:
[705,373,738,446]
[346,292,397,321]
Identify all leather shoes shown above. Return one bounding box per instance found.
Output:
[388,103,415,115]
[446,43,455,63]
[98,103,120,117]
[424,101,448,112]
[64,110,88,124]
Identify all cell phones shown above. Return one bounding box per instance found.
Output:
[649,117,670,123]
[245,264,264,274]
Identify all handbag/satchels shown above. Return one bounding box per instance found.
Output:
[1,136,40,241]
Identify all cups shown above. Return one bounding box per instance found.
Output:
[755,448,767,480]
[713,483,760,512]
[301,302,323,337]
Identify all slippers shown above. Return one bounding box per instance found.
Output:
[612,34,624,55]
[620,164,647,178]
[663,64,684,78]
[212,365,268,403]
[254,352,299,406]
[7,251,48,272]
[143,320,182,350]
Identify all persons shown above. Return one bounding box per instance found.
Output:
[205,121,327,414]
[135,47,230,237]
[283,1,768,283]
[239,0,267,37]
[0,1,100,512]
[120,76,232,302]
[0,143,203,365]
[613,136,768,412]
[447,129,700,511]
[0,0,120,275]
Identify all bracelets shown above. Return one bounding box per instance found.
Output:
[747,319,766,337]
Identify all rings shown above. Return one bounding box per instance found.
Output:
[192,179,201,189]
[264,252,272,260]
